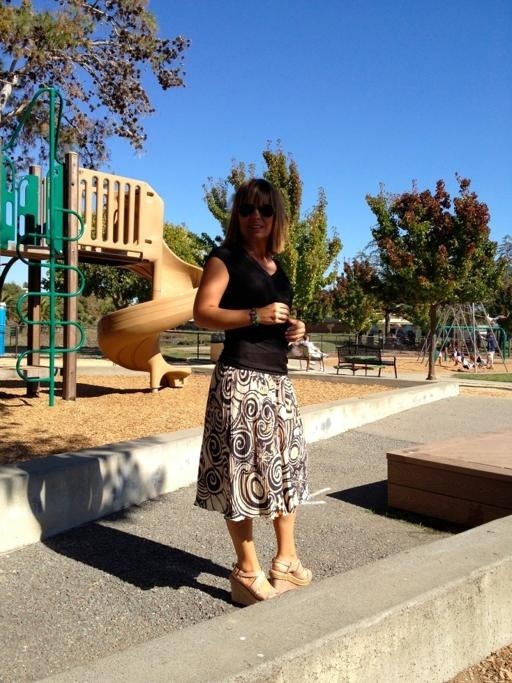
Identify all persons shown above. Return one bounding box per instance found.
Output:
[356,323,418,351]
[190,177,314,606]
[481,313,507,322]
[300,334,330,358]
[433,328,496,372]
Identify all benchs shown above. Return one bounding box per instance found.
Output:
[335,344,397,378]
[286,344,324,372]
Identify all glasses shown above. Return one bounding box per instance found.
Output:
[236,203,274,217]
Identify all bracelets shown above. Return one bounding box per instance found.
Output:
[248,306,261,328]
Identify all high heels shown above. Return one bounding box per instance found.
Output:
[228,562,279,605]
[269,557,312,592]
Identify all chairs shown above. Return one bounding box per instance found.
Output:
[387,337,426,350]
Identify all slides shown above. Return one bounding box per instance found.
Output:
[98,241,205,392]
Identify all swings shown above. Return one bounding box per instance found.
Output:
[449,302,490,371]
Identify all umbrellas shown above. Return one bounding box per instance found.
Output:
[373,312,413,324]
